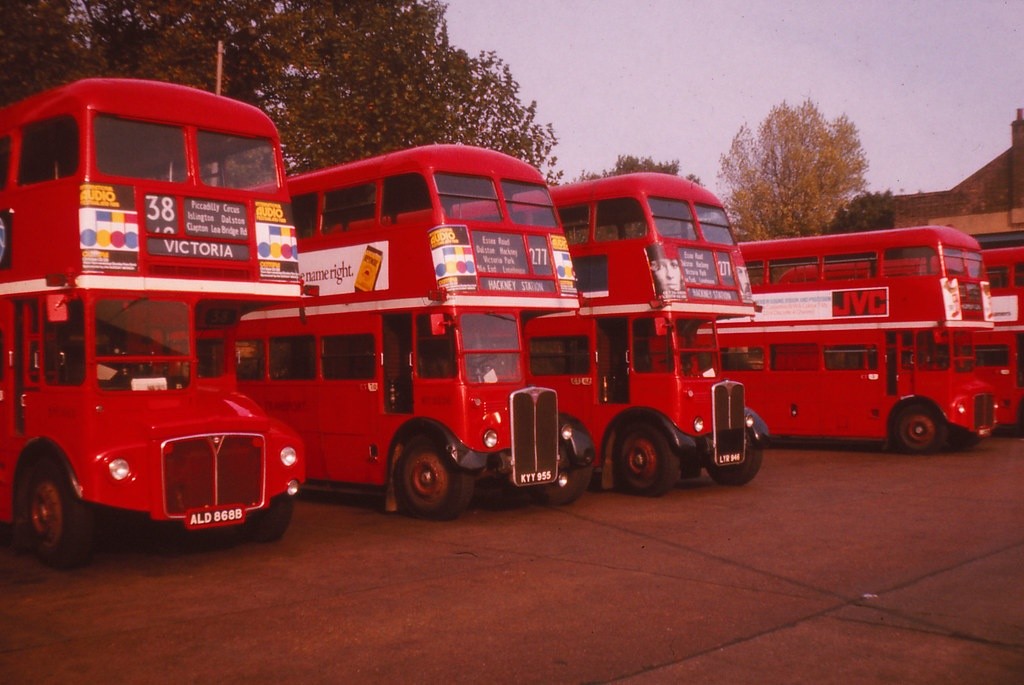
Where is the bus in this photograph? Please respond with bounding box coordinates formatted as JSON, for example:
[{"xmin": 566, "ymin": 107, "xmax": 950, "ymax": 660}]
[
  {"xmin": 778, "ymin": 245, "xmax": 1022, "ymax": 441},
  {"xmin": 2, "ymin": 72, "xmax": 313, "ymax": 570},
  {"xmin": 220, "ymin": 145, "xmax": 601, "ymax": 523},
  {"xmin": 334, "ymin": 170, "xmax": 772, "ymax": 498},
  {"xmin": 700, "ymin": 219, "xmax": 999, "ymax": 455}
]
